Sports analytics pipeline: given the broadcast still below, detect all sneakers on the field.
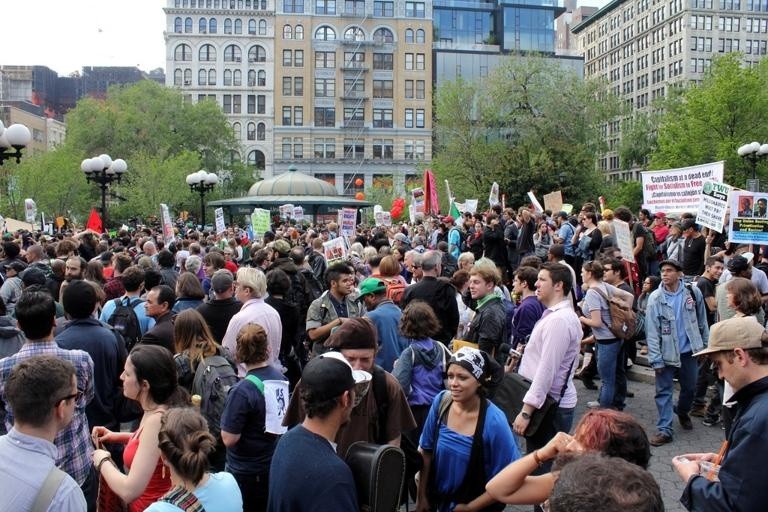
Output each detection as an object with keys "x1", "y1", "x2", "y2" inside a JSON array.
[{"x1": 581, "y1": 378, "x2": 723, "y2": 447}]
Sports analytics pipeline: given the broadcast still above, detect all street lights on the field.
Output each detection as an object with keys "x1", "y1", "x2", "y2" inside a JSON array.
[
  {"x1": 81, "y1": 152, "x2": 130, "y2": 234},
  {"x1": 737, "y1": 140, "x2": 767, "y2": 266},
  {"x1": 186, "y1": 169, "x2": 218, "y2": 228},
  {"x1": 1, "y1": 118, "x2": 30, "y2": 167}
]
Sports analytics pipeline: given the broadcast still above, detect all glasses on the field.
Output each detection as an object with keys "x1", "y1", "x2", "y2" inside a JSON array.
[
  {"x1": 604, "y1": 268, "x2": 614, "y2": 272},
  {"x1": 54, "y1": 390, "x2": 84, "y2": 409}
]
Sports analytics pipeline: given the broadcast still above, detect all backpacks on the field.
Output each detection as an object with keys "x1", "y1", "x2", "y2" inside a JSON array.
[
  {"x1": 592, "y1": 286, "x2": 637, "y2": 340},
  {"x1": 107, "y1": 299, "x2": 143, "y2": 349},
  {"x1": 192, "y1": 353, "x2": 240, "y2": 434}
]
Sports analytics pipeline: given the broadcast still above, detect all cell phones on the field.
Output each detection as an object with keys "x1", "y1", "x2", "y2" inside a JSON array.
[{"x1": 678, "y1": 456, "x2": 690, "y2": 464}]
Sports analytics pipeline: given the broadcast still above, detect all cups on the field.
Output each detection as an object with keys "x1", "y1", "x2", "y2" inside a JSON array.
[{"x1": 699, "y1": 459, "x2": 723, "y2": 482}]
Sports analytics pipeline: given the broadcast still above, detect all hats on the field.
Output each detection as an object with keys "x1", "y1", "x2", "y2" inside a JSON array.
[
  {"x1": 268, "y1": 239, "x2": 291, "y2": 254},
  {"x1": 658, "y1": 259, "x2": 683, "y2": 271},
  {"x1": 5, "y1": 262, "x2": 46, "y2": 288},
  {"x1": 355, "y1": 278, "x2": 385, "y2": 301},
  {"x1": 691, "y1": 318, "x2": 764, "y2": 356},
  {"x1": 302, "y1": 350, "x2": 373, "y2": 393},
  {"x1": 726, "y1": 252, "x2": 755, "y2": 273}
]
